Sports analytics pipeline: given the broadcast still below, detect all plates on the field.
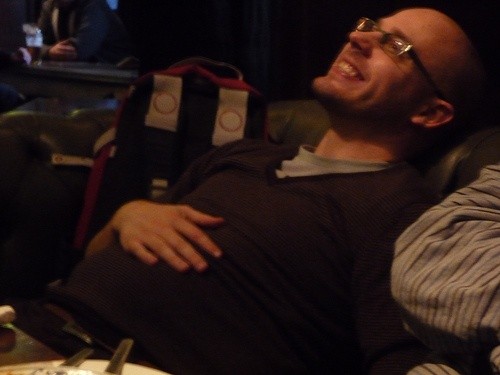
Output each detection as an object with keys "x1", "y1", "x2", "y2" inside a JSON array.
[{"x1": 2, "y1": 359, "x2": 169, "y2": 375}]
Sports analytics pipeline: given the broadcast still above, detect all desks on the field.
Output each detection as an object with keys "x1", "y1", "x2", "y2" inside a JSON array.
[
  {"x1": 0, "y1": 322, "x2": 66, "y2": 374},
  {"x1": 22, "y1": 60, "x2": 138, "y2": 104}
]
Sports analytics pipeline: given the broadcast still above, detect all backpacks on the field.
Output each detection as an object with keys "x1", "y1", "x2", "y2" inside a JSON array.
[{"x1": 76, "y1": 55, "x2": 272, "y2": 253}]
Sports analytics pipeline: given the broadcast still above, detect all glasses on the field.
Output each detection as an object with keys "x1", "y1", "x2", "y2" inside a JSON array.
[{"x1": 355, "y1": 16, "x2": 448, "y2": 103}]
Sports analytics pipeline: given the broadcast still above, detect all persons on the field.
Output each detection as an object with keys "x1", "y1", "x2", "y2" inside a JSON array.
[
  {"x1": 19, "y1": 1, "x2": 486, "y2": 375},
  {"x1": 25, "y1": 0, "x2": 121, "y2": 131}
]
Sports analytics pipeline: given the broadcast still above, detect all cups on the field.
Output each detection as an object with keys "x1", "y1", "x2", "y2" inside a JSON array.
[{"x1": 26, "y1": 36, "x2": 43, "y2": 66}]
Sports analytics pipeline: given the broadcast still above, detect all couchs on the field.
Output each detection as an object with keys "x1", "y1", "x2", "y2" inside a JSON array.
[{"x1": 1, "y1": 103, "x2": 500, "y2": 311}]
